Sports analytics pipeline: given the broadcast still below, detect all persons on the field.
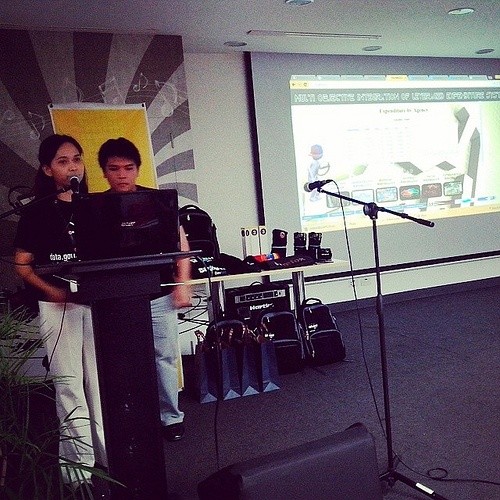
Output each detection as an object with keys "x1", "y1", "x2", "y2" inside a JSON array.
[
  {"x1": 96, "y1": 137, "x2": 193, "y2": 441},
  {"x1": 14, "y1": 133, "x2": 112, "y2": 500}
]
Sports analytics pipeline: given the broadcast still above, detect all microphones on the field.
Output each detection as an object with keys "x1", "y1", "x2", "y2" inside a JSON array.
[
  {"x1": 70, "y1": 175, "x2": 80, "y2": 195},
  {"x1": 304, "y1": 180, "x2": 333, "y2": 192}
]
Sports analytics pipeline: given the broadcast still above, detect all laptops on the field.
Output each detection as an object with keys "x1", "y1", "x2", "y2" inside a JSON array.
[{"x1": 72, "y1": 189, "x2": 182, "y2": 262}]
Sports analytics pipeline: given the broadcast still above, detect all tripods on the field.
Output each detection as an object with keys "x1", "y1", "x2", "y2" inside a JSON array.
[{"x1": 318, "y1": 189, "x2": 449, "y2": 500}]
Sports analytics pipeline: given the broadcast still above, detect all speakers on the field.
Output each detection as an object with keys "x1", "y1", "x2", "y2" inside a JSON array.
[
  {"x1": 198, "y1": 422, "x2": 384, "y2": 500},
  {"x1": 225, "y1": 283, "x2": 289, "y2": 324}
]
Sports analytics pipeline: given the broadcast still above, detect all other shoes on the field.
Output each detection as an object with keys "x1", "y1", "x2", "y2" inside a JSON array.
[
  {"x1": 75, "y1": 482, "x2": 97, "y2": 500},
  {"x1": 162, "y1": 422, "x2": 185, "y2": 441}
]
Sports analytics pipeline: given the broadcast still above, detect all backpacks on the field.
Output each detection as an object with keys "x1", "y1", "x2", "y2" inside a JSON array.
[
  {"x1": 300, "y1": 298, "x2": 346, "y2": 366},
  {"x1": 255, "y1": 302, "x2": 304, "y2": 375},
  {"x1": 178, "y1": 205, "x2": 220, "y2": 279},
  {"x1": 204, "y1": 310, "x2": 248, "y2": 348}
]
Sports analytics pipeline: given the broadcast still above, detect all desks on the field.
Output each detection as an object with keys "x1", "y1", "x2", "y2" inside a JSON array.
[{"x1": 190, "y1": 259, "x2": 349, "y2": 360}]
